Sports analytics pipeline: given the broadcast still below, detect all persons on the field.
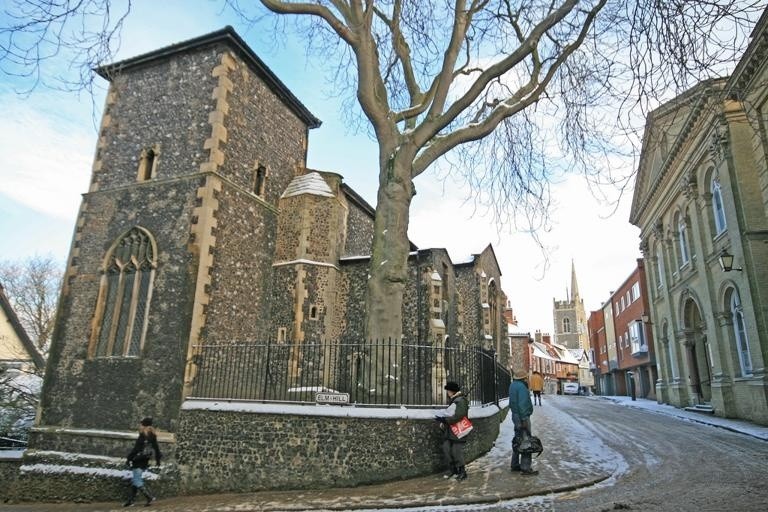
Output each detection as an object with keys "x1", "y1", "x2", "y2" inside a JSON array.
[
  {"x1": 530, "y1": 369, "x2": 545, "y2": 405},
  {"x1": 122, "y1": 416, "x2": 163, "y2": 506},
  {"x1": 434, "y1": 381, "x2": 470, "y2": 482},
  {"x1": 509, "y1": 368, "x2": 540, "y2": 475}
]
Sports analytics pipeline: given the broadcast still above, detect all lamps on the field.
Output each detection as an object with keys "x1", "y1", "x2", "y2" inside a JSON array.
[{"x1": 718, "y1": 247, "x2": 743, "y2": 272}]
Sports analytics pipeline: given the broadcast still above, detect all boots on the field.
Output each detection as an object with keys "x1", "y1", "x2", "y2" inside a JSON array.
[
  {"x1": 124, "y1": 486, "x2": 136, "y2": 507},
  {"x1": 138, "y1": 484, "x2": 155, "y2": 507},
  {"x1": 456, "y1": 466, "x2": 466, "y2": 481},
  {"x1": 449, "y1": 463, "x2": 457, "y2": 479}
]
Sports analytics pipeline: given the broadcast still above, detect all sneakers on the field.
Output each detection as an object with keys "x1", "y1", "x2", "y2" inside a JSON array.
[
  {"x1": 510, "y1": 467, "x2": 520, "y2": 471},
  {"x1": 521, "y1": 469, "x2": 538, "y2": 476}
]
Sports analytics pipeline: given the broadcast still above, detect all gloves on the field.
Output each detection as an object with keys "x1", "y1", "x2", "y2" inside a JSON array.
[{"x1": 434, "y1": 416, "x2": 446, "y2": 423}]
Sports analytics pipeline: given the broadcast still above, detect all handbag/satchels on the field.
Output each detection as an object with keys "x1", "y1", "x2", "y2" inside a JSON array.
[
  {"x1": 132, "y1": 455, "x2": 148, "y2": 469},
  {"x1": 433, "y1": 421, "x2": 449, "y2": 442},
  {"x1": 512, "y1": 436, "x2": 543, "y2": 454}
]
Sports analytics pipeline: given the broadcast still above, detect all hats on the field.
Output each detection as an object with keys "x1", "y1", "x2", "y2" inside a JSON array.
[
  {"x1": 513, "y1": 369, "x2": 528, "y2": 379},
  {"x1": 445, "y1": 382, "x2": 459, "y2": 392},
  {"x1": 141, "y1": 418, "x2": 153, "y2": 426}
]
